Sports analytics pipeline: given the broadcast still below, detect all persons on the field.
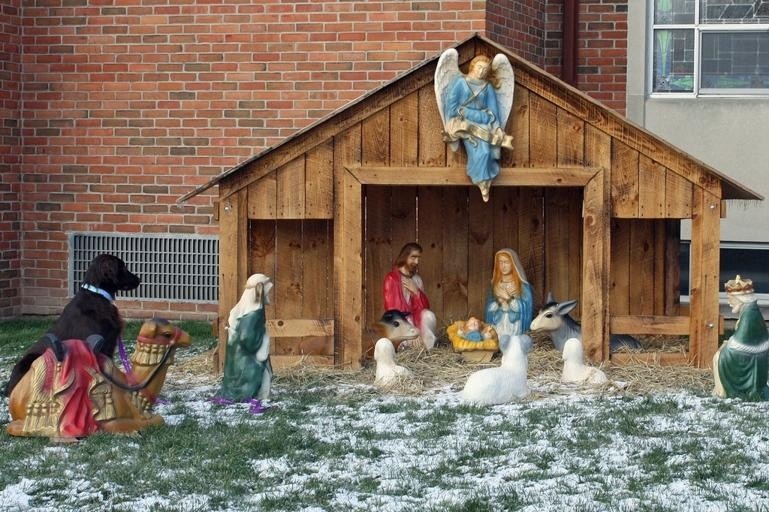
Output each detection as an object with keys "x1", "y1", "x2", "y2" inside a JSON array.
[
  {"x1": 382, "y1": 240, "x2": 439, "y2": 353},
  {"x1": 440, "y1": 54, "x2": 505, "y2": 204},
  {"x1": 459, "y1": 316, "x2": 492, "y2": 342},
  {"x1": 486, "y1": 248, "x2": 535, "y2": 355},
  {"x1": 710, "y1": 298, "x2": 769, "y2": 405},
  {"x1": 210, "y1": 272, "x2": 278, "y2": 407}
]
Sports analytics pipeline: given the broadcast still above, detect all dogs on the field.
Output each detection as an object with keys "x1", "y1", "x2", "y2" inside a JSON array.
[{"x1": 3, "y1": 254, "x2": 143, "y2": 398}]
